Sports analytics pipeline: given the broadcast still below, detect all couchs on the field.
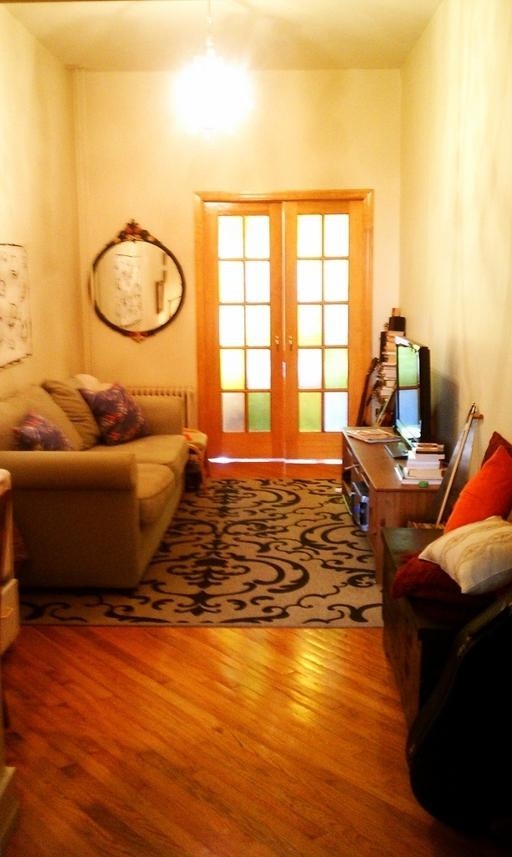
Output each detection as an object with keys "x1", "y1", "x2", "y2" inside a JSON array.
[{"x1": 0, "y1": 384, "x2": 190, "y2": 590}]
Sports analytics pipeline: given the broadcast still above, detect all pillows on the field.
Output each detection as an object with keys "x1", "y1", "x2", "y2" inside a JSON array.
[
  {"x1": 14, "y1": 373, "x2": 141, "y2": 451},
  {"x1": 388, "y1": 443, "x2": 512, "y2": 608}
]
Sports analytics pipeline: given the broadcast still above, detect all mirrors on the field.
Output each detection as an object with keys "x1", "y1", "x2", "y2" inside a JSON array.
[{"x1": 84, "y1": 219, "x2": 185, "y2": 343}]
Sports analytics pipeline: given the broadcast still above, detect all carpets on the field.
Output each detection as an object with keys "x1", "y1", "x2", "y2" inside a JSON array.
[{"x1": 20, "y1": 477, "x2": 385, "y2": 631}]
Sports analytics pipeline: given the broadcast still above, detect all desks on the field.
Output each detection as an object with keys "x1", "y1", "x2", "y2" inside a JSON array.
[{"x1": 339, "y1": 426, "x2": 445, "y2": 553}]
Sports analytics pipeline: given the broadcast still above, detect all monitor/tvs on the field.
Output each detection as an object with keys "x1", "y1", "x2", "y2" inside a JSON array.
[{"x1": 383, "y1": 335, "x2": 431, "y2": 459}]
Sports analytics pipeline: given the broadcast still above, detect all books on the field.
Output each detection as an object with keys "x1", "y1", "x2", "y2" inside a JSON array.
[
  {"x1": 394, "y1": 443, "x2": 445, "y2": 484},
  {"x1": 346, "y1": 429, "x2": 401, "y2": 443},
  {"x1": 381, "y1": 331, "x2": 404, "y2": 395}
]
